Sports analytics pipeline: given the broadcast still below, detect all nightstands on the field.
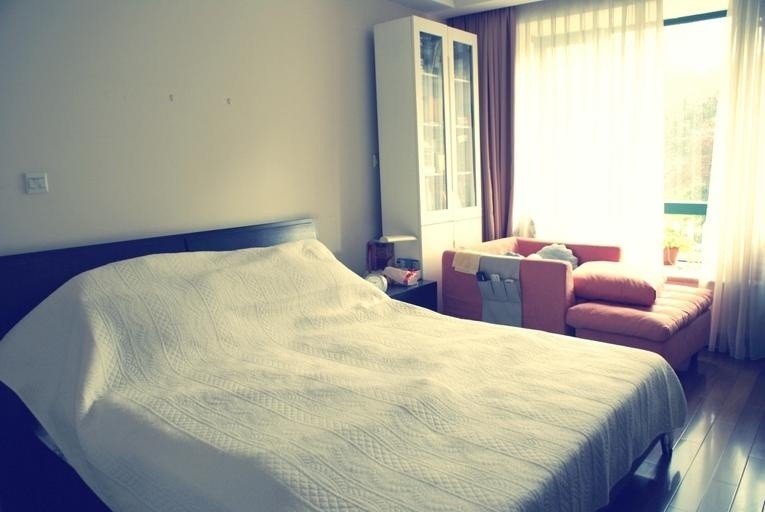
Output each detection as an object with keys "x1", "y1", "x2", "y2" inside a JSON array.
[{"x1": 355, "y1": 270, "x2": 437, "y2": 312}]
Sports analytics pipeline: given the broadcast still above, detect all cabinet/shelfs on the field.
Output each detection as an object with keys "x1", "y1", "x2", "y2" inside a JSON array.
[{"x1": 373, "y1": 14, "x2": 485, "y2": 310}]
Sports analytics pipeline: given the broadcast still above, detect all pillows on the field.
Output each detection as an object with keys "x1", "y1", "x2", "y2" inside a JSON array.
[
  {"x1": 573, "y1": 260, "x2": 666, "y2": 305},
  {"x1": 536, "y1": 243, "x2": 579, "y2": 270}
]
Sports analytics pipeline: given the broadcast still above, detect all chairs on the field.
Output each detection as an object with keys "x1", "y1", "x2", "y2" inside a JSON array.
[{"x1": 442, "y1": 236, "x2": 620, "y2": 345}]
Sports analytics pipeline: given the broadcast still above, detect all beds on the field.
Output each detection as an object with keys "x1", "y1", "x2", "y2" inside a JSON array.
[{"x1": 0, "y1": 215, "x2": 671, "y2": 512}]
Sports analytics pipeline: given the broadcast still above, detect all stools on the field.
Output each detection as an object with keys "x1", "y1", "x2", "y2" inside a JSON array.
[{"x1": 566, "y1": 282, "x2": 714, "y2": 371}]
